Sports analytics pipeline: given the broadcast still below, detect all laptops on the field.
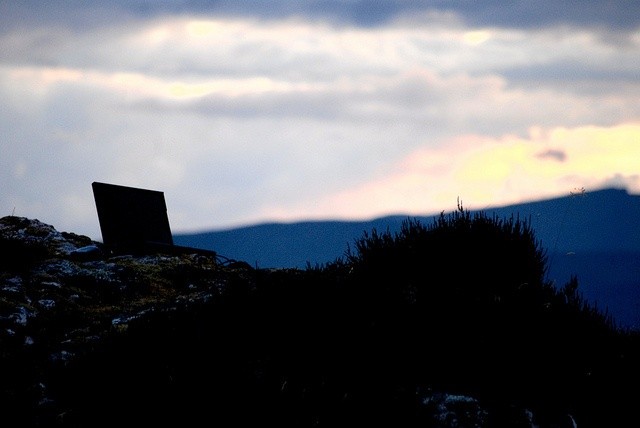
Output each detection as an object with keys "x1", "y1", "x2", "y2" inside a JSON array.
[{"x1": 92, "y1": 182, "x2": 216, "y2": 258}]
